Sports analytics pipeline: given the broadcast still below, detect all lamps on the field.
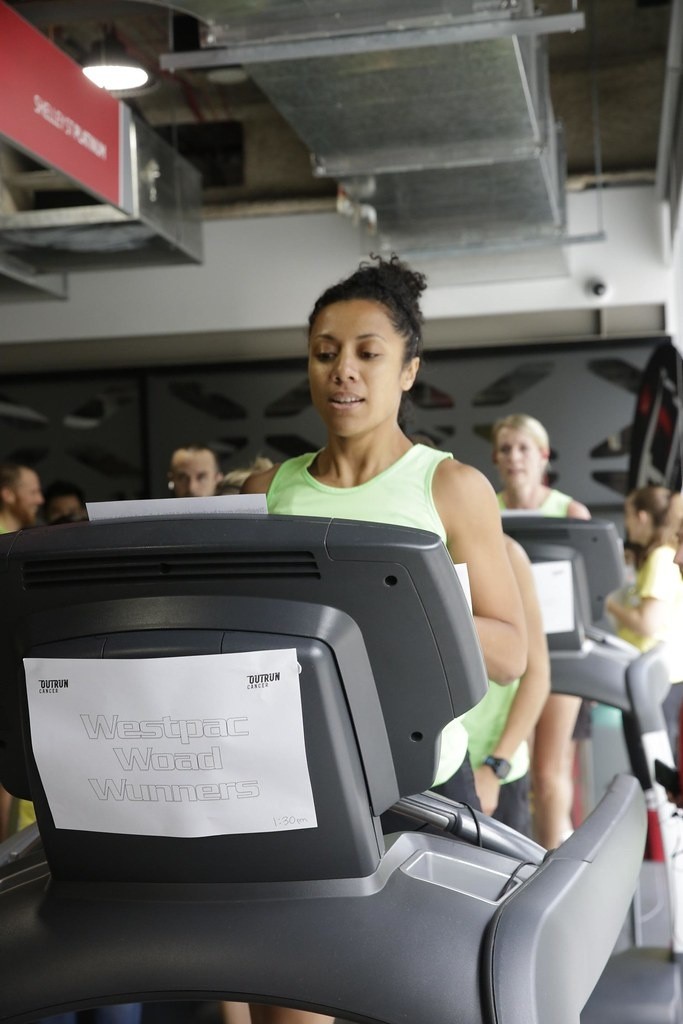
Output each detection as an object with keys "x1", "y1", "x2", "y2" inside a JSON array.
[{"x1": 80, "y1": 20, "x2": 152, "y2": 91}]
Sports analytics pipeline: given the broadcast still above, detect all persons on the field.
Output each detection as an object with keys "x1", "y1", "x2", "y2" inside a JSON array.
[
  {"x1": 221, "y1": 253, "x2": 527, "y2": 1024},
  {"x1": 171, "y1": 447, "x2": 218, "y2": 496},
  {"x1": 411, "y1": 435, "x2": 550, "y2": 839},
  {"x1": 605, "y1": 485, "x2": 683, "y2": 817},
  {"x1": 44, "y1": 480, "x2": 85, "y2": 524},
  {"x1": 0, "y1": 463, "x2": 43, "y2": 841},
  {"x1": 496, "y1": 414, "x2": 597, "y2": 843}
]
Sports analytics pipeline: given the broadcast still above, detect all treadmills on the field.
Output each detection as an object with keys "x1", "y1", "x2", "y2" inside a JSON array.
[{"x1": 0, "y1": 506, "x2": 682, "y2": 1024}]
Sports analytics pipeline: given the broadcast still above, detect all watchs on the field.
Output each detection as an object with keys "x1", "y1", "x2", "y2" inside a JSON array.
[{"x1": 483, "y1": 755, "x2": 512, "y2": 779}]
